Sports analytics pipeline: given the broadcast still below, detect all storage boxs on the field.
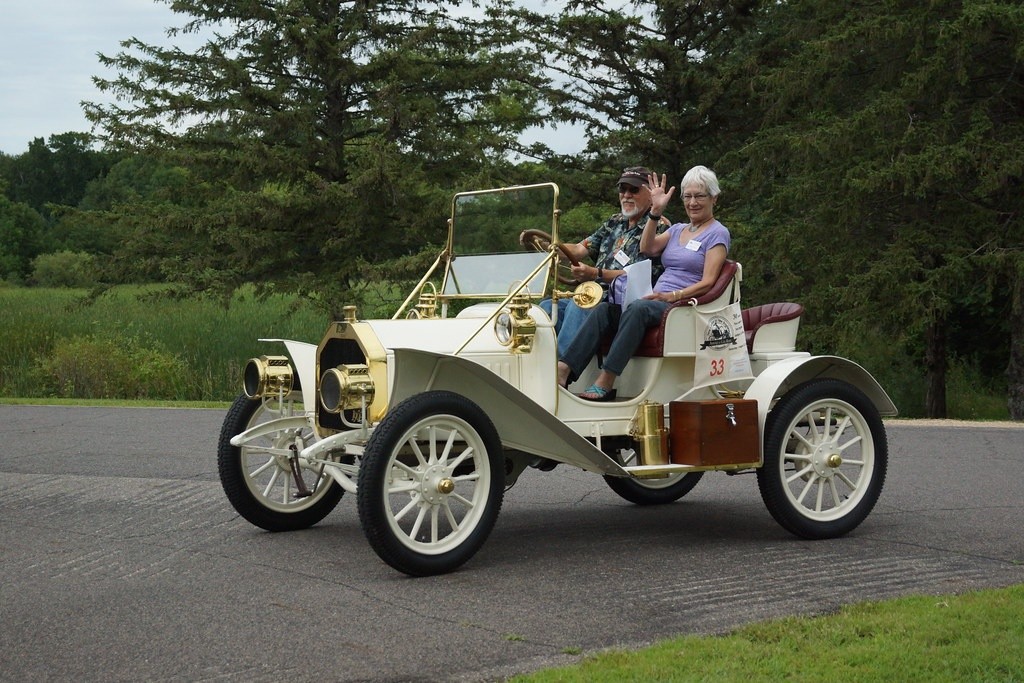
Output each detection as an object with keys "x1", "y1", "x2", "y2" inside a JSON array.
[{"x1": 669, "y1": 397, "x2": 759, "y2": 466}]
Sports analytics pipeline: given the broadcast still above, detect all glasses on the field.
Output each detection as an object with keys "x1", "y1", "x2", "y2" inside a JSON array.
[
  {"x1": 619, "y1": 185, "x2": 640, "y2": 193},
  {"x1": 681, "y1": 193, "x2": 711, "y2": 201}
]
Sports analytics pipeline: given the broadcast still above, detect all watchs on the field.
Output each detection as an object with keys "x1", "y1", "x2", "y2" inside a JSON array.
[{"x1": 595, "y1": 266, "x2": 603, "y2": 283}]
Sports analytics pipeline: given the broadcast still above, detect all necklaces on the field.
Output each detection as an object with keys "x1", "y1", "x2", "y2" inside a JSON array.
[{"x1": 688, "y1": 216, "x2": 715, "y2": 233}]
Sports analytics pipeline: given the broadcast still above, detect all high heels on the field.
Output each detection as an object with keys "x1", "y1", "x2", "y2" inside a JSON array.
[{"x1": 577, "y1": 383, "x2": 617, "y2": 402}]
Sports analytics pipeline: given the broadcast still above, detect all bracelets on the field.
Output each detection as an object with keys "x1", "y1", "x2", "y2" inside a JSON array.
[
  {"x1": 680, "y1": 289, "x2": 684, "y2": 300},
  {"x1": 648, "y1": 212, "x2": 661, "y2": 221},
  {"x1": 672, "y1": 291, "x2": 676, "y2": 301}
]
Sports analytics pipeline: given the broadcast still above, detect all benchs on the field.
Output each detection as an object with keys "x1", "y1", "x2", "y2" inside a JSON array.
[{"x1": 596, "y1": 257, "x2": 742, "y2": 358}]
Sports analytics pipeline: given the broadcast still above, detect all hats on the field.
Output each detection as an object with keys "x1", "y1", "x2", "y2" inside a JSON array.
[{"x1": 615, "y1": 166, "x2": 652, "y2": 188}]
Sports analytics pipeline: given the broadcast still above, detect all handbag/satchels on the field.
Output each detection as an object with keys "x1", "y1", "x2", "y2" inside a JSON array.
[{"x1": 608, "y1": 274, "x2": 627, "y2": 304}]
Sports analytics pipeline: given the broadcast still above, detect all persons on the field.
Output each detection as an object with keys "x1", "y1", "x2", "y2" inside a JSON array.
[{"x1": 519, "y1": 164, "x2": 731, "y2": 401}]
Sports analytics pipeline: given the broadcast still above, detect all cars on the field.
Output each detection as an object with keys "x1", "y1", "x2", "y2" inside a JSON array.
[{"x1": 215, "y1": 182, "x2": 900, "y2": 577}]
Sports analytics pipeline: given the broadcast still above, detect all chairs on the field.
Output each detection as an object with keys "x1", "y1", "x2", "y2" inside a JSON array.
[{"x1": 741, "y1": 303, "x2": 804, "y2": 354}]
